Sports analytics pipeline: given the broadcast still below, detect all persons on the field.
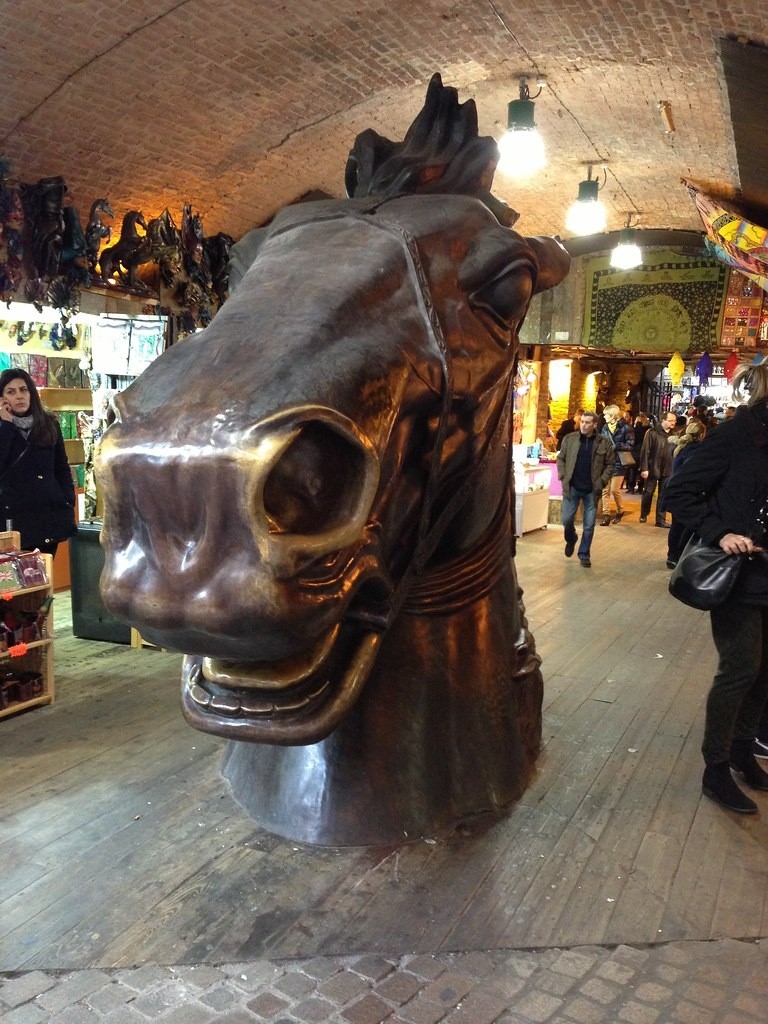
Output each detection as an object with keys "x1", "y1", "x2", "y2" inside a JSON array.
[
  {"x1": 555, "y1": 403, "x2": 737, "y2": 568},
  {"x1": 0, "y1": 368, "x2": 79, "y2": 559},
  {"x1": 156, "y1": 203, "x2": 214, "y2": 339},
  {"x1": 662, "y1": 356, "x2": 768, "y2": 812}
]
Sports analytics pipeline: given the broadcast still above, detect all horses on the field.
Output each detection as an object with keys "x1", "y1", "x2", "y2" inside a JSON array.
[{"x1": 92, "y1": 74, "x2": 571, "y2": 845}]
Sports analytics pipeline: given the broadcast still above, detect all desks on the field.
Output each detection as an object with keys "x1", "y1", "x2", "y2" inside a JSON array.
[
  {"x1": 67, "y1": 522, "x2": 131, "y2": 646},
  {"x1": 0, "y1": 553, "x2": 54, "y2": 718}
]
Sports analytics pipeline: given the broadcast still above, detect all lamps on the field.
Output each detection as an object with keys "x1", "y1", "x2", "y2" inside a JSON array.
[
  {"x1": 566, "y1": 166, "x2": 607, "y2": 235},
  {"x1": 609, "y1": 212, "x2": 643, "y2": 269},
  {"x1": 498, "y1": 84, "x2": 547, "y2": 177}
]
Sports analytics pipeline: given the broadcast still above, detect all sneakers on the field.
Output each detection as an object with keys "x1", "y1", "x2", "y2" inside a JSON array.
[{"x1": 752, "y1": 737, "x2": 768, "y2": 760}]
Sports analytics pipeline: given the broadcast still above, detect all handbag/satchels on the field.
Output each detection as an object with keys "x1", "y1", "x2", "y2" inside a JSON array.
[
  {"x1": 617, "y1": 451, "x2": 636, "y2": 465},
  {"x1": 667, "y1": 532, "x2": 747, "y2": 612}
]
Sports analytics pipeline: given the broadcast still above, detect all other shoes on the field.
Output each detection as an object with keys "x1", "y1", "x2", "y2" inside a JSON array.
[
  {"x1": 625, "y1": 489, "x2": 634, "y2": 494},
  {"x1": 665, "y1": 560, "x2": 677, "y2": 569},
  {"x1": 702, "y1": 761, "x2": 758, "y2": 813},
  {"x1": 634, "y1": 488, "x2": 643, "y2": 495},
  {"x1": 612, "y1": 512, "x2": 624, "y2": 524},
  {"x1": 728, "y1": 740, "x2": 768, "y2": 790},
  {"x1": 600, "y1": 515, "x2": 610, "y2": 526}
]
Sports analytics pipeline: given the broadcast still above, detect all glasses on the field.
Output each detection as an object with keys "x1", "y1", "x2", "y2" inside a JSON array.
[{"x1": 666, "y1": 419, "x2": 677, "y2": 423}]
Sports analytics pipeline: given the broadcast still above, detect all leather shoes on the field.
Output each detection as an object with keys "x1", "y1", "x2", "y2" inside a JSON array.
[
  {"x1": 655, "y1": 521, "x2": 672, "y2": 528},
  {"x1": 565, "y1": 536, "x2": 578, "y2": 557},
  {"x1": 580, "y1": 559, "x2": 591, "y2": 568},
  {"x1": 639, "y1": 513, "x2": 647, "y2": 522}
]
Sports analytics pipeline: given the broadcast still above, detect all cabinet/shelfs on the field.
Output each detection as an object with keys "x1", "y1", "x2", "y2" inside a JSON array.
[
  {"x1": 515, "y1": 465, "x2": 552, "y2": 538},
  {"x1": 33, "y1": 385, "x2": 94, "y2": 594}
]
